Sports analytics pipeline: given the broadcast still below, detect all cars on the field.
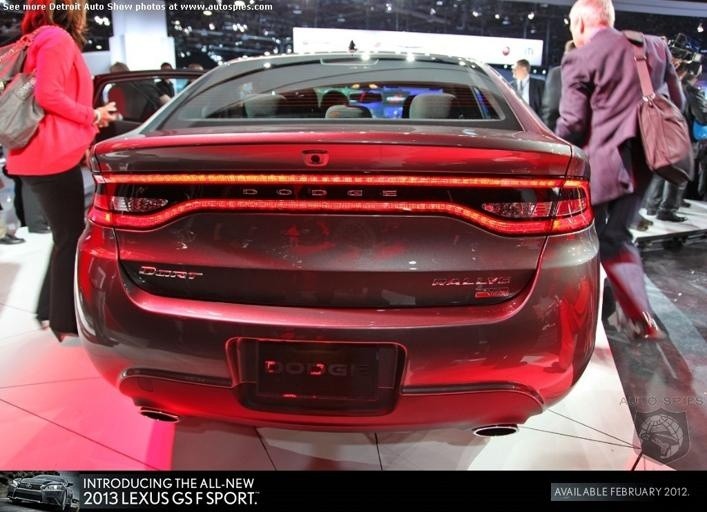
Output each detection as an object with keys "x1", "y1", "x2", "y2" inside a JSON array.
[
  {"x1": 0, "y1": 145, "x2": 98, "y2": 243},
  {"x1": 64, "y1": 42, "x2": 606, "y2": 442}
]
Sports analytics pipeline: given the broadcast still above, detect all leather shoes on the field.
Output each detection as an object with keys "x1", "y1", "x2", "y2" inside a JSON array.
[
  {"x1": 628, "y1": 313, "x2": 661, "y2": 342},
  {"x1": 630, "y1": 198, "x2": 690, "y2": 232},
  {"x1": 0, "y1": 233, "x2": 24, "y2": 245},
  {"x1": 28, "y1": 222, "x2": 52, "y2": 233}
]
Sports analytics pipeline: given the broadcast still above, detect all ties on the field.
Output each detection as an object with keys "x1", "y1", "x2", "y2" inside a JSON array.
[{"x1": 518, "y1": 82, "x2": 523, "y2": 97}]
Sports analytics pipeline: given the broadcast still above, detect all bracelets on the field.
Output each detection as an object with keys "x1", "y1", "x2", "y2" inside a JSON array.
[{"x1": 92, "y1": 110, "x2": 102, "y2": 126}]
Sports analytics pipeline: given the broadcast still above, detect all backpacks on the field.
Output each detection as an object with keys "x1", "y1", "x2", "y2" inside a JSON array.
[{"x1": 636, "y1": 95, "x2": 694, "y2": 183}]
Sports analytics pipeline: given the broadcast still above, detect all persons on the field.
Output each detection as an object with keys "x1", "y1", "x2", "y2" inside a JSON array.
[
  {"x1": 0, "y1": 145, "x2": 25, "y2": 246},
  {"x1": 553, "y1": 0, "x2": 688, "y2": 339},
  {"x1": 107, "y1": 63, "x2": 151, "y2": 124},
  {"x1": 16, "y1": 175, "x2": 52, "y2": 234},
  {"x1": 507, "y1": 59, "x2": 547, "y2": 123},
  {"x1": 0, "y1": 1, "x2": 121, "y2": 345},
  {"x1": 628, "y1": 33, "x2": 706, "y2": 231},
  {"x1": 154, "y1": 63, "x2": 176, "y2": 108},
  {"x1": 183, "y1": 63, "x2": 205, "y2": 90}
]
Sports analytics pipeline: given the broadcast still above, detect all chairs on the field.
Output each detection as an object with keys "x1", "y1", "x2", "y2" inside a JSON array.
[{"x1": 200, "y1": 93, "x2": 461, "y2": 119}]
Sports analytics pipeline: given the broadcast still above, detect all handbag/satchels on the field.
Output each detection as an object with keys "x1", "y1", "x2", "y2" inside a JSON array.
[{"x1": 0, "y1": 73, "x2": 45, "y2": 151}]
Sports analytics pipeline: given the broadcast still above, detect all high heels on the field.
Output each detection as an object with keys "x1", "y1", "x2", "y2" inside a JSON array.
[
  {"x1": 49, "y1": 320, "x2": 82, "y2": 341},
  {"x1": 36, "y1": 314, "x2": 60, "y2": 330}
]
